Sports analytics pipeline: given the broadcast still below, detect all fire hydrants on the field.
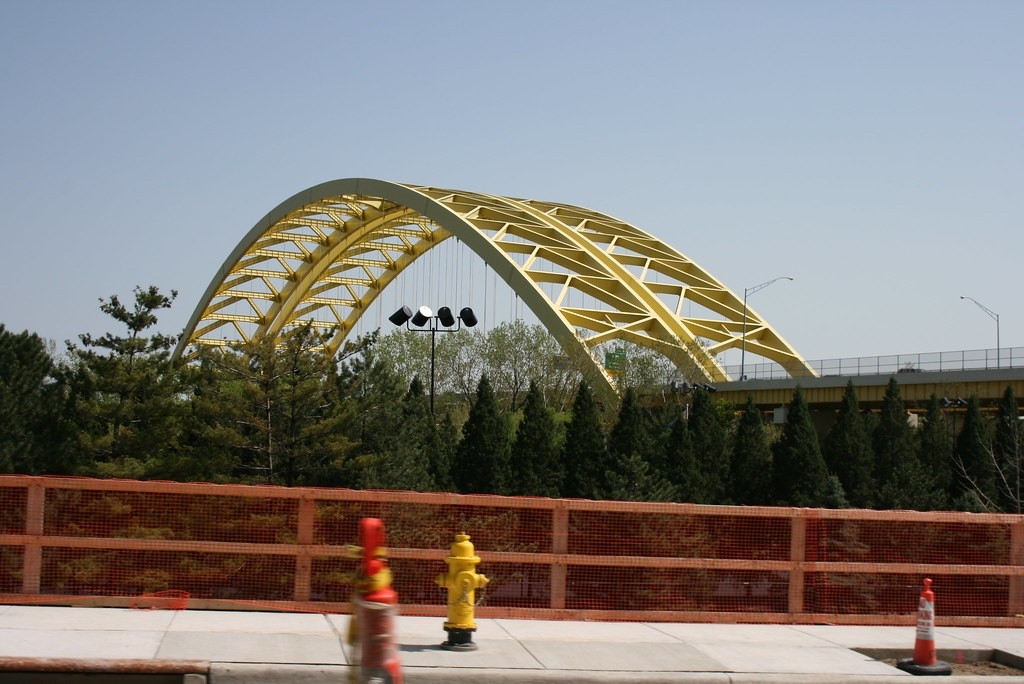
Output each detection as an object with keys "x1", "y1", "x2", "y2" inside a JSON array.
[{"x1": 431, "y1": 529, "x2": 492, "y2": 653}]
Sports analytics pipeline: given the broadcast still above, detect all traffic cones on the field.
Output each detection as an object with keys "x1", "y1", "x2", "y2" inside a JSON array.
[{"x1": 895, "y1": 577, "x2": 953, "y2": 676}]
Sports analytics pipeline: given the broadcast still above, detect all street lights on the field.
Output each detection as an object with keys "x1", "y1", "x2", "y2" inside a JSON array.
[
  {"x1": 388, "y1": 305, "x2": 478, "y2": 412},
  {"x1": 739, "y1": 275, "x2": 795, "y2": 382},
  {"x1": 960, "y1": 295, "x2": 1000, "y2": 368}
]
